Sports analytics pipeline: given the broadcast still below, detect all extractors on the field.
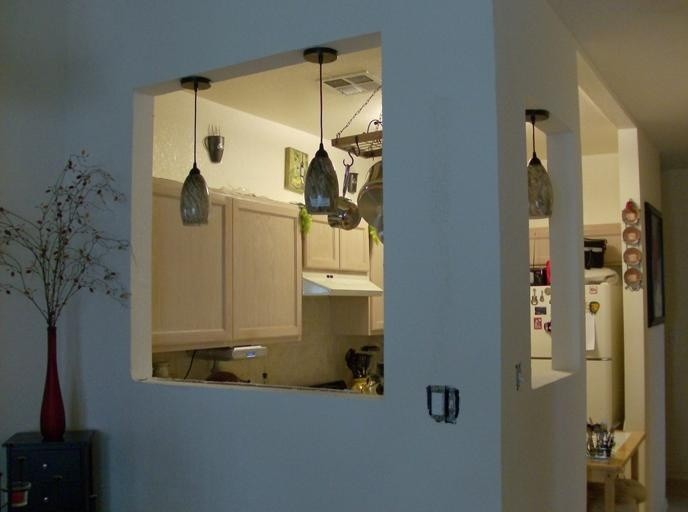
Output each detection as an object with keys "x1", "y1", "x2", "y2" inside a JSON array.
[{"x1": 304, "y1": 274, "x2": 383, "y2": 298}]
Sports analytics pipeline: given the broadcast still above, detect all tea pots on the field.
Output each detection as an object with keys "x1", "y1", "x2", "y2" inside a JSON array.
[{"x1": 348, "y1": 375, "x2": 371, "y2": 392}]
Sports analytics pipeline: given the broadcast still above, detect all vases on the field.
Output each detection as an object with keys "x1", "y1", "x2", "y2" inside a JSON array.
[{"x1": 39, "y1": 328, "x2": 66, "y2": 442}]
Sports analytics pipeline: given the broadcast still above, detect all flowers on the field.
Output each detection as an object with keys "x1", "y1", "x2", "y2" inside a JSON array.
[{"x1": 0, "y1": 148, "x2": 135, "y2": 328}]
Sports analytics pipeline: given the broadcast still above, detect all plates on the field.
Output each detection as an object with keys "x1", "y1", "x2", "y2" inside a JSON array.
[
  {"x1": 622, "y1": 209, "x2": 641, "y2": 224},
  {"x1": 623, "y1": 248, "x2": 642, "y2": 265},
  {"x1": 624, "y1": 269, "x2": 641, "y2": 286},
  {"x1": 623, "y1": 226, "x2": 640, "y2": 244}
]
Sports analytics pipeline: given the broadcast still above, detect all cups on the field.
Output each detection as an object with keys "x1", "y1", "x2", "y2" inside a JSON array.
[{"x1": 349, "y1": 173, "x2": 358, "y2": 193}]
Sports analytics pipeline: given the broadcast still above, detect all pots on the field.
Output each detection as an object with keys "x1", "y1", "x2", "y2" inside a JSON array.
[
  {"x1": 357, "y1": 161, "x2": 383, "y2": 229},
  {"x1": 328, "y1": 165, "x2": 363, "y2": 230}
]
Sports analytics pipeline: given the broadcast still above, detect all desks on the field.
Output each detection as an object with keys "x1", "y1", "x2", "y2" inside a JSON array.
[{"x1": 586, "y1": 430, "x2": 647, "y2": 512}]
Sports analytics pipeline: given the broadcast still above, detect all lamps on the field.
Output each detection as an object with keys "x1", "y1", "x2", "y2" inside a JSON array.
[
  {"x1": 179, "y1": 77, "x2": 211, "y2": 228},
  {"x1": 524, "y1": 107, "x2": 554, "y2": 221},
  {"x1": 301, "y1": 47, "x2": 340, "y2": 214}
]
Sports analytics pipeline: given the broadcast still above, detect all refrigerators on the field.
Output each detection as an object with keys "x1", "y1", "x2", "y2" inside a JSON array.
[{"x1": 530, "y1": 284, "x2": 622, "y2": 431}]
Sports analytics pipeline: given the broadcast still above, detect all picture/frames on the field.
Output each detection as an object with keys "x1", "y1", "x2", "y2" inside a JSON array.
[{"x1": 644, "y1": 199, "x2": 667, "y2": 328}]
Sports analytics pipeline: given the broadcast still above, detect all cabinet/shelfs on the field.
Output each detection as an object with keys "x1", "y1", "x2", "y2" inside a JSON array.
[
  {"x1": 149, "y1": 175, "x2": 304, "y2": 355},
  {"x1": 3, "y1": 428, "x2": 97, "y2": 512},
  {"x1": 302, "y1": 204, "x2": 371, "y2": 276},
  {"x1": 331, "y1": 226, "x2": 384, "y2": 338}
]
